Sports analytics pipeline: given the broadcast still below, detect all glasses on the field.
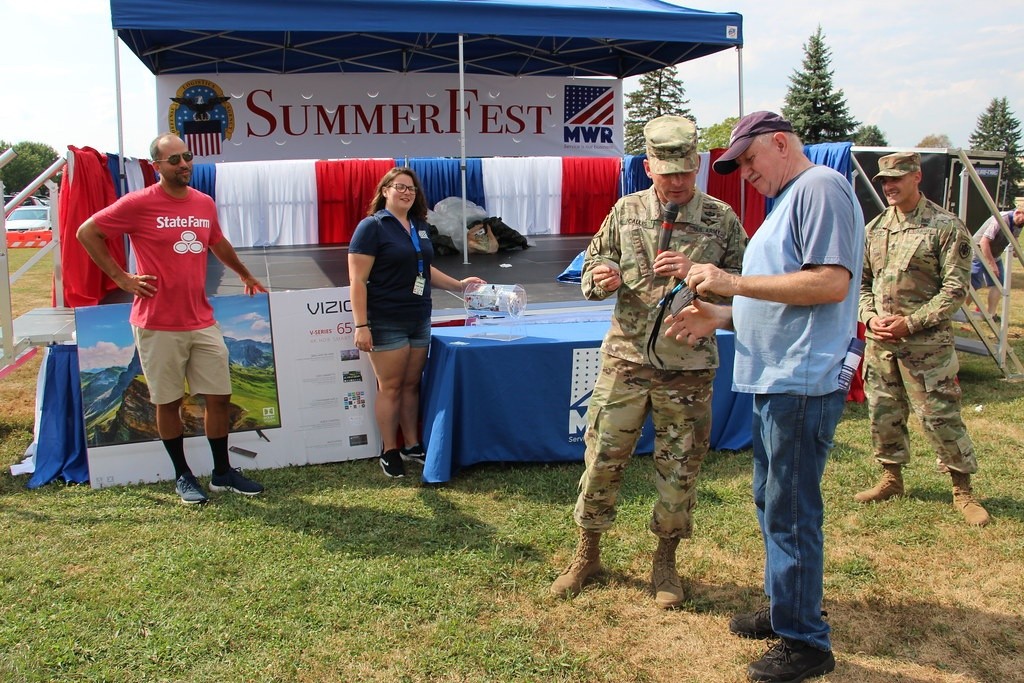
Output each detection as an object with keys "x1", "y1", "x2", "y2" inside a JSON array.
[
  {"x1": 388, "y1": 183, "x2": 418, "y2": 195},
  {"x1": 157, "y1": 151, "x2": 193, "y2": 165}
]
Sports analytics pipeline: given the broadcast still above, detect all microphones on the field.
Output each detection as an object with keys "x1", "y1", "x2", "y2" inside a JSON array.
[{"x1": 657, "y1": 201, "x2": 679, "y2": 257}]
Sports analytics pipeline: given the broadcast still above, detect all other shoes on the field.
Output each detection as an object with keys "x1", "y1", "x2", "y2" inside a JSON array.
[{"x1": 984, "y1": 313, "x2": 1001, "y2": 323}]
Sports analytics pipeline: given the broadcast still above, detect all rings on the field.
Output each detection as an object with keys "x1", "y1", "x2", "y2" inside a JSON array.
[{"x1": 671, "y1": 264, "x2": 675, "y2": 271}]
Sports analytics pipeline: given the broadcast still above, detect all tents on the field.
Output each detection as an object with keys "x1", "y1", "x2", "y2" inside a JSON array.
[
  {"x1": 109, "y1": 0, "x2": 744, "y2": 273},
  {"x1": 348, "y1": 166, "x2": 487, "y2": 478}
]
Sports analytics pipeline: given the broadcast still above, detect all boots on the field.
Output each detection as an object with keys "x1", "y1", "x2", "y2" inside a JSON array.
[
  {"x1": 855, "y1": 464, "x2": 904, "y2": 503},
  {"x1": 551, "y1": 526, "x2": 602, "y2": 598},
  {"x1": 648, "y1": 537, "x2": 685, "y2": 609},
  {"x1": 951, "y1": 470, "x2": 990, "y2": 527}
]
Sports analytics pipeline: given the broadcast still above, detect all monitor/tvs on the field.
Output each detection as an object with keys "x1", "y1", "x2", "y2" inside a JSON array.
[{"x1": 75, "y1": 293, "x2": 282, "y2": 442}]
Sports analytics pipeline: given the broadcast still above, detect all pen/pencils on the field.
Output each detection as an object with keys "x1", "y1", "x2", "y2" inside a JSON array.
[
  {"x1": 656, "y1": 278, "x2": 687, "y2": 309},
  {"x1": 478, "y1": 315, "x2": 506, "y2": 319}
]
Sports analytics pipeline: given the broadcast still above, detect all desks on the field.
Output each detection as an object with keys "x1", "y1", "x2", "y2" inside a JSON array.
[{"x1": 420, "y1": 297, "x2": 753, "y2": 482}]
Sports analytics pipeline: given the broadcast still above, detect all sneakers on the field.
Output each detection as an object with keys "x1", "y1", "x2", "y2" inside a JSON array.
[
  {"x1": 400, "y1": 444, "x2": 426, "y2": 465},
  {"x1": 748, "y1": 636, "x2": 836, "y2": 683},
  {"x1": 208, "y1": 467, "x2": 265, "y2": 495},
  {"x1": 379, "y1": 448, "x2": 405, "y2": 479},
  {"x1": 729, "y1": 606, "x2": 828, "y2": 639},
  {"x1": 175, "y1": 472, "x2": 206, "y2": 504}
]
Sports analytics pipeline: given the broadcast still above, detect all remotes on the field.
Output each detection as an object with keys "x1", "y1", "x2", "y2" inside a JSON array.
[{"x1": 229, "y1": 446, "x2": 257, "y2": 458}]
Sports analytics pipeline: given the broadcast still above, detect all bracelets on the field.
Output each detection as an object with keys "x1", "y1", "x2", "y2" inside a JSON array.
[
  {"x1": 461, "y1": 280, "x2": 464, "y2": 293},
  {"x1": 356, "y1": 323, "x2": 368, "y2": 328}
]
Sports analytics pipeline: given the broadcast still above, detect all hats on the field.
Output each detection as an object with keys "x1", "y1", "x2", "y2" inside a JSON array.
[
  {"x1": 712, "y1": 111, "x2": 794, "y2": 175},
  {"x1": 643, "y1": 114, "x2": 699, "y2": 175},
  {"x1": 872, "y1": 152, "x2": 922, "y2": 181}
]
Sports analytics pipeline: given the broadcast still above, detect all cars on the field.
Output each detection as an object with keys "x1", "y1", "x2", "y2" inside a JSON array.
[
  {"x1": 4, "y1": 193, "x2": 49, "y2": 206},
  {"x1": 5, "y1": 206, "x2": 52, "y2": 232}
]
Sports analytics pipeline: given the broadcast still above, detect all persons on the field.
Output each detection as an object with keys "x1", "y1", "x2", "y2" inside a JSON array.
[
  {"x1": 966, "y1": 197, "x2": 1024, "y2": 323},
  {"x1": 687, "y1": 111, "x2": 866, "y2": 683},
  {"x1": 551, "y1": 116, "x2": 750, "y2": 609},
  {"x1": 76, "y1": 132, "x2": 268, "y2": 504},
  {"x1": 858, "y1": 152, "x2": 990, "y2": 526}
]
